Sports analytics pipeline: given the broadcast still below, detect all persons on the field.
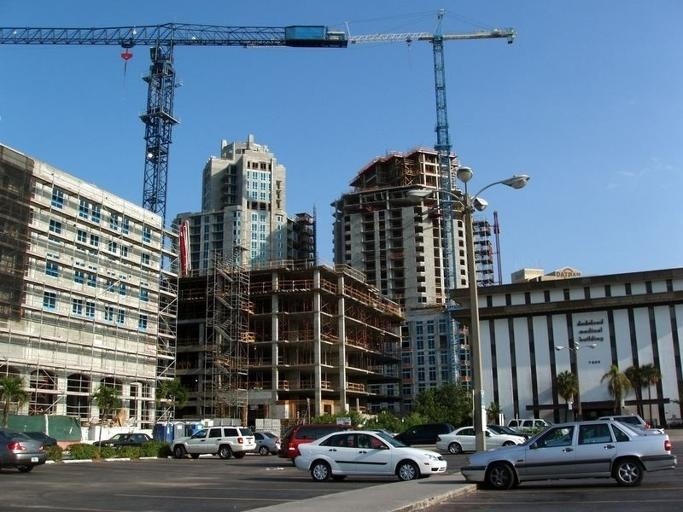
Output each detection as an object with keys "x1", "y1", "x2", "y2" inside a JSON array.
[{"x1": 360, "y1": 436, "x2": 374, "y2": 448}]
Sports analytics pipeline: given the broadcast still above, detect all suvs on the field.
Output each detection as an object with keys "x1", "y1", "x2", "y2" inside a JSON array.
[
  {"x1": 279, "y1": 425, "x2": 387, "y2": 466},
  {"x1": 393, "y1": 423, "x2": 455, "y2": 447},
  {"x1": 506, "y1": 419, "x2": 549, "y2": 433},
  {"x1": 170, "y1": 426, "x2": 257, "y2": 460}
]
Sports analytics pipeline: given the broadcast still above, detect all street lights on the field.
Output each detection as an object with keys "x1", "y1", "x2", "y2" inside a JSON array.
[
  {"x1": 406, "y1": 165, "x2": 530, "y2": 452},
  {"x1": 555, "y1": 342, "x2": 597, "y2": 422}
]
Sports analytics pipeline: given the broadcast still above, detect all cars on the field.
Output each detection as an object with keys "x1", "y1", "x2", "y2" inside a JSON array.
[
  {"x1": 460, "y1": 420, "x2": 678, "y2": 490},
  {"x1": 246, "y1": 431, "x2": 282, "y2": 457},
  {"x1": 547, "y1": 415, "x2": 666, "y2": 447},
  {"x1": 0, "y1": 428, "x2": 57, "y2": 473},
  {"x1": 295, "y1": 430, "x2": 447, "y2": 483},
  {"x1": 438, "y1": 425, "x2": 527, "y2": 454},
  {"x1": 93, "y1": 433, "x2": 153, "y2": 448},
  {"x1": 487, "y1": 425, "x2": 532, "y2": 440}
]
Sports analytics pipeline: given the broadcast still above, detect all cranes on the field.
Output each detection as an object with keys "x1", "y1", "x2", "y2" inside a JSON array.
[
  {"x1": 0, "y1": 23, "x2": 348, "y2": 285},
  {"x1": 327, "y1": 8, "x2": 517, "y2": 384}
]
